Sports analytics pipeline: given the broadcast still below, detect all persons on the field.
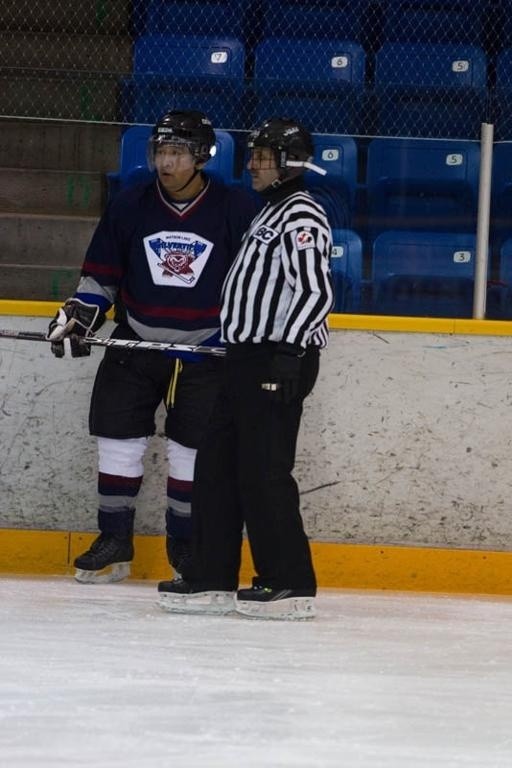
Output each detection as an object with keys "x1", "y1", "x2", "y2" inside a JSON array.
[
  {"x1": 46, "y1": 110, "x2": 262, "y2": 572},
  {"x1": 158, "y1": 114, "x2": 335, "y2": 601}
]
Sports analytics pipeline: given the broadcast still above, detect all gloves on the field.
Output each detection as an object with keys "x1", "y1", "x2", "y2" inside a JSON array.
[
  {"x1": 45, "y1": 297, "x2": 106, "y2": 359},
  {"x1": 260, "y1": 340, "x2": 306, "y2": 406}
]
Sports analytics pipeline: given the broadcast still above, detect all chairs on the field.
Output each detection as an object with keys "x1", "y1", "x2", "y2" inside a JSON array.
[{"x1": 100, "y1": 2, "x2": 510, "y2": 318}]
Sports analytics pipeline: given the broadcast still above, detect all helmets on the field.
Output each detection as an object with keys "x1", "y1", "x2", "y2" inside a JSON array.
[
  {"x1": 242, "y1": 116, "x2": 315, "y2": 179},
  {"x1": 144, "y1": 109, "x2": 216, "y2": 175}
]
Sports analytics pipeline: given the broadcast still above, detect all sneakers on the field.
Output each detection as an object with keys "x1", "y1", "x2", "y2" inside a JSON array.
[
  {"x1": 73, "y1": 531, "x2": 134, "y2": 571},
  {"x1": 165, "y1": 534, "x2": 193, "y2": 575},
  {"x1": 158, "y1": 576, "x2": 239, "y2": 594},
  {"x1": 237, "y1": 584, "x2": 316, "y2": 602}
]
